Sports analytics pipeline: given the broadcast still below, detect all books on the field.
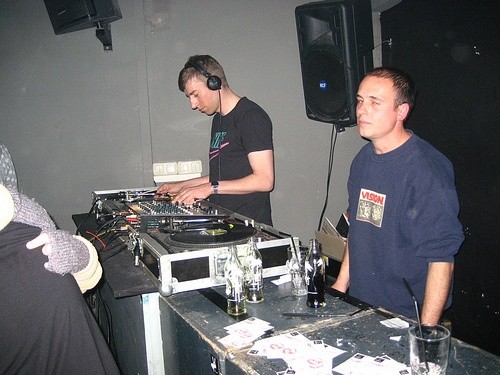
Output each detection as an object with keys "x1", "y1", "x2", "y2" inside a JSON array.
[{"x1": 320, "y1": 212, "x2": 350, "y2": 240}]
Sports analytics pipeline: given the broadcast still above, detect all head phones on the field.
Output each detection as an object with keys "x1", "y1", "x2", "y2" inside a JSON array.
[{"x1": 187, "y1": 60, "x2": 221, "y2": 90}]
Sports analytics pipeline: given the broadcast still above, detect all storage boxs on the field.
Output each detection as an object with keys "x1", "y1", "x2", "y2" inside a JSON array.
[{"x1": 314, "y1": 230, "x2": 348, "y2": 261}]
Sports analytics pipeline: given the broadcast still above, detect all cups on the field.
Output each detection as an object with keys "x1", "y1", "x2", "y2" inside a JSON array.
[
  {"x1": 408, "y1": 323, "x2": 451, "y2": 375},
  {"x1": 287, "y1": 246, "x2": 310, "y2": 296}
]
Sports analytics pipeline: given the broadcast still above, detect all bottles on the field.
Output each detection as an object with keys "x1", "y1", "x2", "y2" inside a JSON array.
[
  {"x1": 304, "y1": 239, "x2": 326, "y2": 308},
  {"x1": 243, "y1": 236, "x2": 265, "y2": 303},
  {"x1": 224, "y1": 245, "x2": 247, "y2": 316}
]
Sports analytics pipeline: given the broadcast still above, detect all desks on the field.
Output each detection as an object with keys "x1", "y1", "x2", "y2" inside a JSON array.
[{"x1": 160, "y1": 277, "x2": 500, "y2": 375}]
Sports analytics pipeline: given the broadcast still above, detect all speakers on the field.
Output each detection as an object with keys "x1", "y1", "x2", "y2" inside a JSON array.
[
  {"x1": 43, "y1": 0, "x2": 121, "y2": 35},
  {"x1": 294, "y1": 0, "x2": 374, "y2": 126}
]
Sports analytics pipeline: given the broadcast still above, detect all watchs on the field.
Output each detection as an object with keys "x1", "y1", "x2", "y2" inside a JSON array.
[{"x1": 211, "y1": 180, "x2": 219, "y2": 194}]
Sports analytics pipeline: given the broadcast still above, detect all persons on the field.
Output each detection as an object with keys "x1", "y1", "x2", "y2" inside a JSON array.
[
  {"x1": 154, "y1": 55, "x2": 274, "y2": 227},
  {"x1": 0, "y1": 142, "x2": 118, "y2": 375},
  {"x1": 329, "y1": 67, "x2": 465, "y2": 327}
]
